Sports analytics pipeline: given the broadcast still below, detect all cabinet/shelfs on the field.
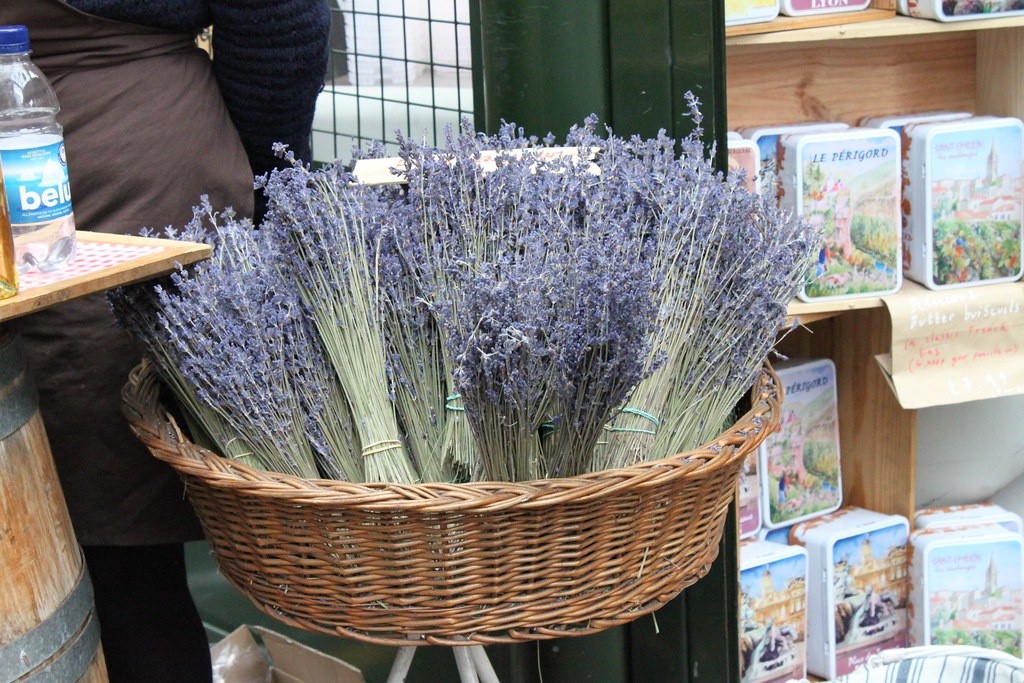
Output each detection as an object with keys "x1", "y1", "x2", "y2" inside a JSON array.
[{"x1": 723, "y1": 0, "x2": 1024, "y2": 683}]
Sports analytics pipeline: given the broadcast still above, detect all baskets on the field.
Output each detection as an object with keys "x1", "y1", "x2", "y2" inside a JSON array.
[{"x1": 119, "y1": 352, "x2": 785, "y2": 646}]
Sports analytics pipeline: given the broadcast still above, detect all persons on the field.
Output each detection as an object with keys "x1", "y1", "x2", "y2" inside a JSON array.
[{"x1": 0, "y1": 0, "x2": 332, "y2": 683}]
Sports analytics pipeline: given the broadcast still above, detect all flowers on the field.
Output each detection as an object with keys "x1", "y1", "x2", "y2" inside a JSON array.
[{"x1": 105, "y1": 90, "x2": 836, "y2": 482}]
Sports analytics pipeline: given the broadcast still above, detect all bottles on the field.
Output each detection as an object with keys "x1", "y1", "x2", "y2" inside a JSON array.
[
  {"x1": 0, "y1": 160, "x2": 20, "y2": 300},
  {"x1": 0, "y1": 24, "x2": 77, "y2": 276}
]
